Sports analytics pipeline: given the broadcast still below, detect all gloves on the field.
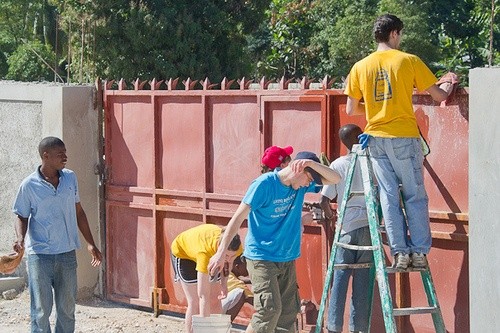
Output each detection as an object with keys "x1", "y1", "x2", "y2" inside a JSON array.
[
  {"x1": 437, "y1": 71, "x2": 458, "y2": 89},
  {"x1": 358, "y1": 134, "x2": 372, "y2": 150}
]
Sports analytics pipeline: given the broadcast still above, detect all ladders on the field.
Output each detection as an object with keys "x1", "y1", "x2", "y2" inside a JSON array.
[{"x1": 314, "y1": 145, "x2": 447, "y2": 333}]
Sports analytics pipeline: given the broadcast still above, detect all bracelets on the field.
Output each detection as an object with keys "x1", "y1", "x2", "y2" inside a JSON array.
[{"x1": 325, "y1": 211, "x2": 336, "y2": 220}]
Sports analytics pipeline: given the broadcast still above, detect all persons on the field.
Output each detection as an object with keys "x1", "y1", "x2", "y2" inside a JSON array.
[
  {"x1": 208, "y1": 151, "x2": 342, "y2": 333},
  {"x1": 217, "y1": 256, "x2": 313, "y2": 326},
  {"x1": 10, "y1": 136, "x2": 103, "y2": 332},
  {"x1": 170, "y1": 224, "x2": 243, "y2": 333},
  {"x1": 259, "y1": 145, "x2": 294, "y2": 177},
  {"x1": 344, "y1": 12, "x2": 460, "y2": 271},
  {"x1": 318, "y1": 124, "x2": 383, "y2": 333}
]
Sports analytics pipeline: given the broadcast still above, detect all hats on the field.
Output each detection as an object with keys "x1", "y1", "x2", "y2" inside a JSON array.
[
  {"x1": 262, "y1": 146, "x2": 293, "y2": 170},
  {"x1": 294, "y1": 151, "x2": 323, "y2": 185}
]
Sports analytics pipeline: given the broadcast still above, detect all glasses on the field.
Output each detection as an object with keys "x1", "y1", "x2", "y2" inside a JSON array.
[
  {"x1": 282, "y1": 156, "x2": 291, "y2": 162},
  {"x1": 305, "y1": 171, "x2": 313, "y2": 183}
]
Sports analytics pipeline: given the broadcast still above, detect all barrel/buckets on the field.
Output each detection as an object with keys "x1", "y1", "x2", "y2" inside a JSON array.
[{"x1": 192, "y1": 314, "x2": 231, "y2": 333}]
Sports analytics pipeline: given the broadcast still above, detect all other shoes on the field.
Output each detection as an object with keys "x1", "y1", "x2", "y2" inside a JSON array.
[
  {"x1": 394, "y1": 252, "x2": 410, "y2": 271},
  {"x1": 412, "y1": 252, "x2": 428, "y2": 271}
]
